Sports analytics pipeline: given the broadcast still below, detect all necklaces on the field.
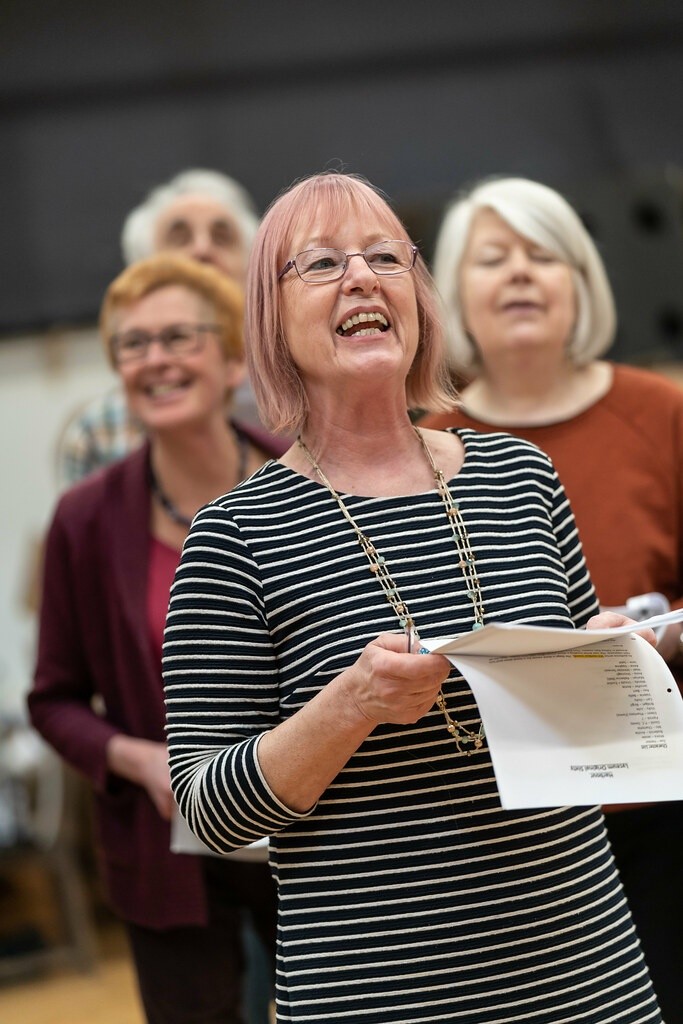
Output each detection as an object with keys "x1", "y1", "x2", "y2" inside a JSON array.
[
  {"x1": 298, "y1": 425, "x2": 487, "y2": 758},
  {"x1": 145, "y1": 426, "x2": 250, "y2": 532}
]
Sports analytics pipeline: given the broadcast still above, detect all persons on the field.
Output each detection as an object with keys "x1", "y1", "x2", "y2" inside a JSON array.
[{"x1": 23, "y1": 167, "x2": 683, "y2": 1024}]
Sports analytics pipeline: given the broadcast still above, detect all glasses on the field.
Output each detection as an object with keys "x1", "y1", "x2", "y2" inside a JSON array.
[
  {"x1": 109, "y1": 322, "x2": 224, "y2": 362},
  {"x1": 278, "y1": 240, "x2": 419, "y2": 283}
]
